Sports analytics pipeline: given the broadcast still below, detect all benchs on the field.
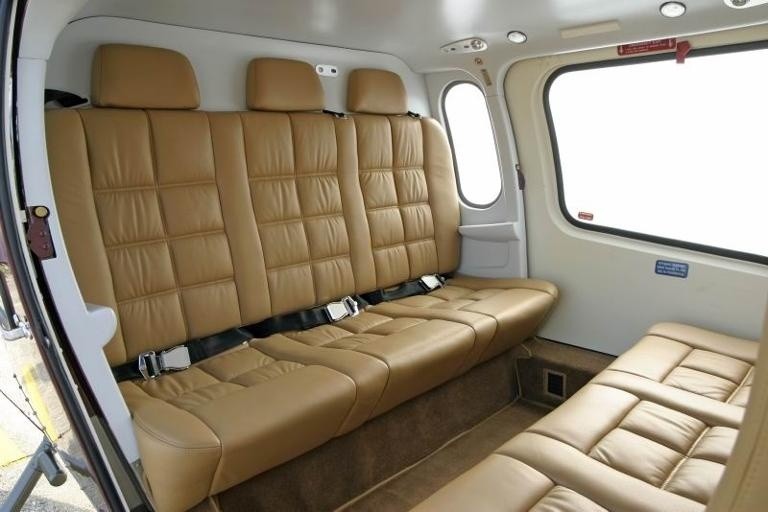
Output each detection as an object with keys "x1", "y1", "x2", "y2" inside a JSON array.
[
  {"x1": 45, "y1": 45, "x2": 558, "y2": 510},
  {"x1": 407, "y1": 299, "x2": 768, "y2": 512}
]
